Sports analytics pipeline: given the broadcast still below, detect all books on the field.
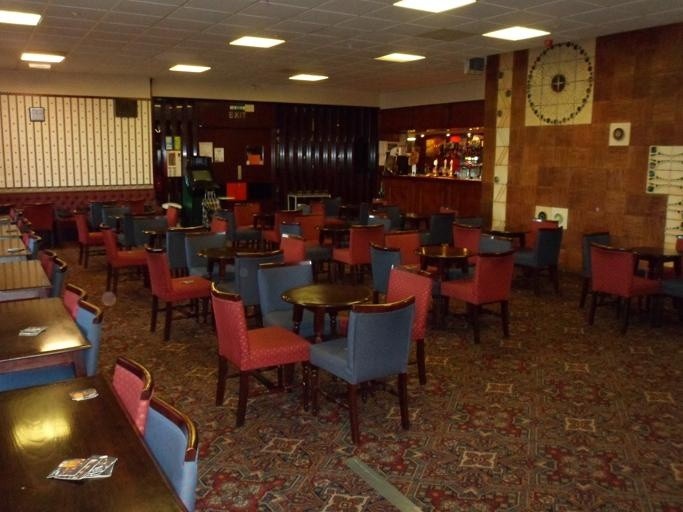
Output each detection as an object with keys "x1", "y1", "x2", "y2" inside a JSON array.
[
  {"x1": 55, "y1": 456, "x2": 90, "y2": 476},
  {"x1": 0, "y1": 217, "x2": 11, "y2": 224},
  {"x1": 19, "y1": 323, "x2": 49, "y2": 338},
  {"x1": 7, "y1": 244, "x2": 27, "y2": 252},
  {"x1": 103, "y1": 464, "x2": 114, "y2": 477},
  {"x1": 66, "y1": 385, "x2": 99, "y2": 402},
  {"x1": 51, "y1": 455, "x2": 119, "y2": 478}
]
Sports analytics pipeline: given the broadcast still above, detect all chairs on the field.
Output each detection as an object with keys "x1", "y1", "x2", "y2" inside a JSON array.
[
  {"x1": 143, "y1": 395, "x2": 198, "y2": 512},
  {"x1": 40, "y1": 249, "x2": 56, "y2": 281},
  {"x1": 63, "y1": 199, "x2": 564, "y2": 445},
  {"x1": 647, "y1": 236, "x2": 683, "y2": 321},
  {"x1": 50, "y1": 258, "x2": 67, "y2": 297},
  {"x1": 588, "y1": 238, "x2": 664, "y2": 337},
  {"x1": 0, "y1": 208, "x2": 42, "y2": 263},
  {"x1": 578, "y1": 232, "x2": 612, "y2": 307}
]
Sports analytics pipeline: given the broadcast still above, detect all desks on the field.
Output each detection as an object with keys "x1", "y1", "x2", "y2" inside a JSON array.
[
  {"x1": 622, "y1": 246, "x2": 683, "y2": 330},
  {"x1": 0, "y1": 260, "x2": 51, "y2": 304}
]
